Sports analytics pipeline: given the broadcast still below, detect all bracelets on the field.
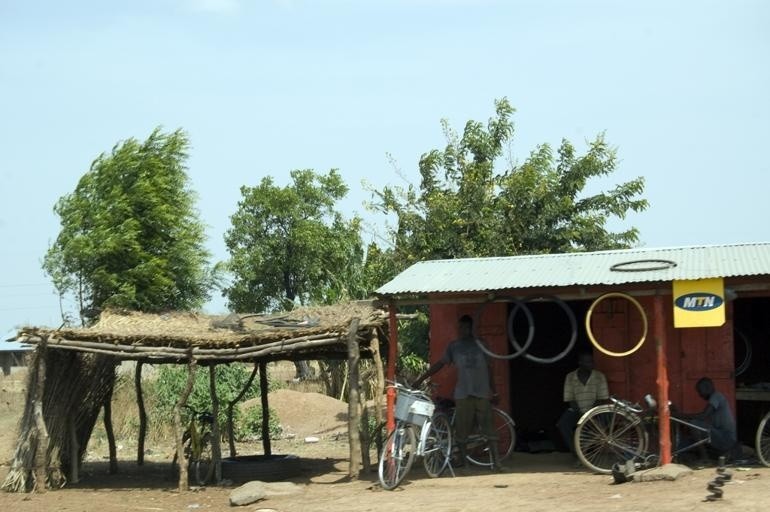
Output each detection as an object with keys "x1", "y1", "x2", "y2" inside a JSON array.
[{"x1": 493, "y1": 391, "x2": 499, "y2": 396}]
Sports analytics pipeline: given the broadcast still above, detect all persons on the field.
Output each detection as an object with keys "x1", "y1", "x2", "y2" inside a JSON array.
[
  {"x1": 556, "y1": 344, "x2": 610, "y2": 468},
  {"x1": 674, "y1": 375, "x2": 738, "y2": 466},
  {"x1": 413, "y1": 313, "x2": 503, "y2": 474}
]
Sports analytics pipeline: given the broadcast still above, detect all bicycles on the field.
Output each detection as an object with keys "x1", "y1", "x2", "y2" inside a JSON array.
[
  {"x1": 379, "y1": 378, "x2": 517, "y2": 491},
  {"x1": 573, "y1": 393, "x2": 714, "y2": 474},
  {"x1": 756, "y1": 410, "x2": 770, "y2": 470},
  {"x1": 168, "y1": 404, "x2": 219, "y2": 483}
]
